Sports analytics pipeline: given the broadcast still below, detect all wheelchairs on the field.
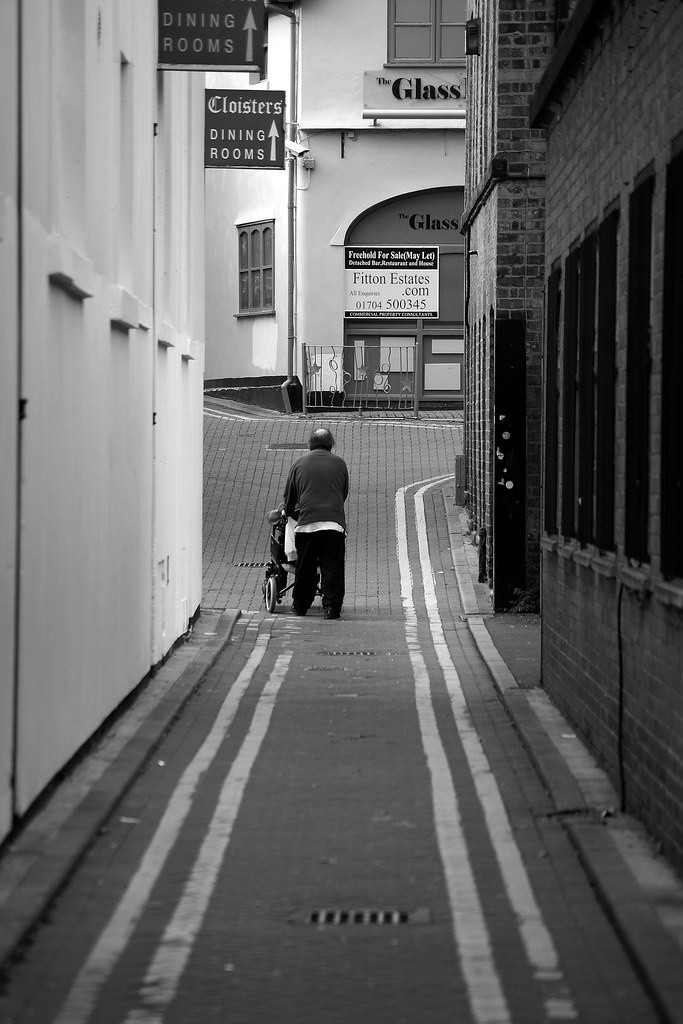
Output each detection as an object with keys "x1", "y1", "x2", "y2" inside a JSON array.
[{"x1": 261, "y1": 509, "x2": 326, "y2": 613}]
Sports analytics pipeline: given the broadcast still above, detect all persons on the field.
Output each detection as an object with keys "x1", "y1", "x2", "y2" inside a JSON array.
[
  {"x1": 262, "y1": 499, "x2": 300, "y2": 602},
  {"x1": 284, "y1": 430, "x2": 349, "y2": 621}
]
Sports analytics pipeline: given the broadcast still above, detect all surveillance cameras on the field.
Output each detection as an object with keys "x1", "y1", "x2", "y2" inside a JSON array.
[{"x1": 285, "y1": 140, "x2": 310, "y2": 158}]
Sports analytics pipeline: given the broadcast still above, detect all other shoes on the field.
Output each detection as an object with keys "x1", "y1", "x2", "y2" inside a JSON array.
[
  {"x1": 291, "y1": 604, "x2": 306, "y2": 616},
  {"x1": 324, "y1": 608, "x2": 341, "y2": 619}
]
